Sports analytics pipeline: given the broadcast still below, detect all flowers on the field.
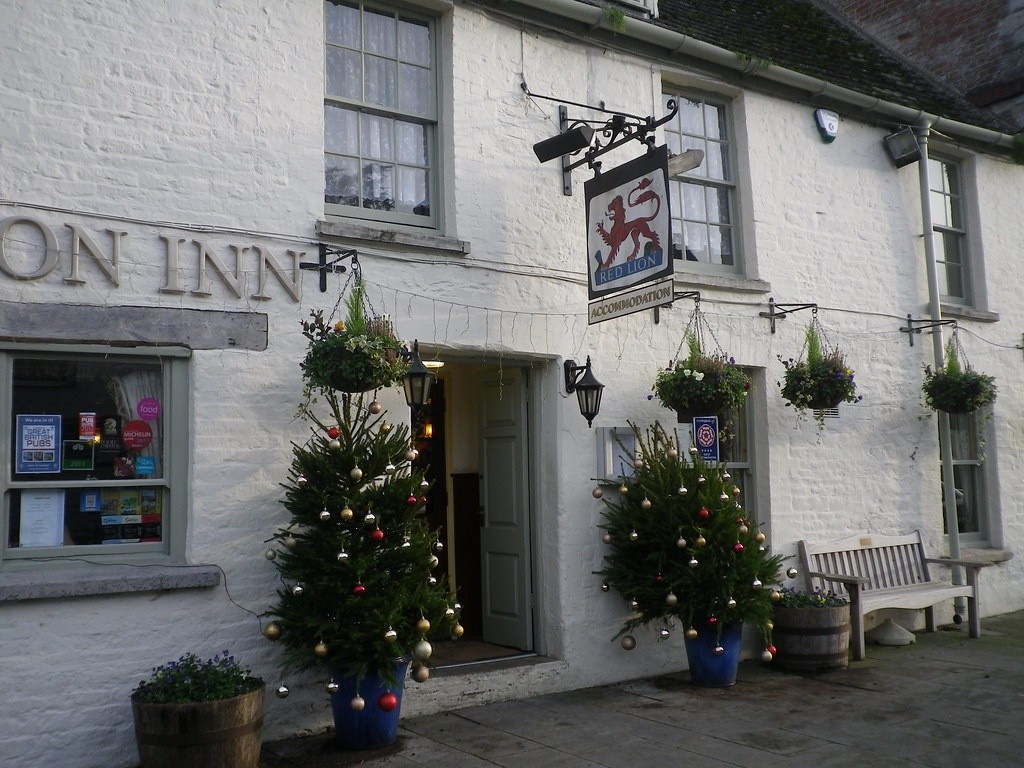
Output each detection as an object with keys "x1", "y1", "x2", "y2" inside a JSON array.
[
  {"x1": 652, "y1": 335, "x2": 749, "y2": 445},
  {"x1": 299, "y1": 311, "x2": 406, "y2": 393},
  {"x1": 919, "y1": 340, "x2": 995, "y2": 445},
  {"x1": 776, "y1": 585, "x2": 846, "y2": 612},
  {"x1": 780, "y1": 333, "x2": 857, "y2": 439},
  {"x1": 142, "y1": 652, "x2": 256, "y2": 703}
]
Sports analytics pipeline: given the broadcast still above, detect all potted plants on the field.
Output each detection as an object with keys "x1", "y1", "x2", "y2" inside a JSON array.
[
  {"x1": 260, "y1": 385, "x2": 467, "y2": 748},
  {"x1": 589, "y1": 417, "x2": 799, "y2": 691}
]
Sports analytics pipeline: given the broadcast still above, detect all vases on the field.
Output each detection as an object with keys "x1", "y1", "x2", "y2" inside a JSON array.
[
  {"x1": 779, "y1": 605, "x2": 851, "y2": 668},
  {"x1": 676, "y1": 384, "x2": 726, "y2": 425},
  {"x1": 929, "y1": 383, "x2": 983, "y2": 415},
  {"x1": 326, "y1": 346, "x2": 400, "y2": 393},
  {"x1": 132, "y1": 681, "x2": 266, "y2": 766},
  {"x1": 803, "y1": 379, "x2": 853, "y2": 411}
]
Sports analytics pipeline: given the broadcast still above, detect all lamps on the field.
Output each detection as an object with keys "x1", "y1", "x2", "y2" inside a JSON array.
[
  {"x1": 885, "y1": 127, "x2": 925, "y2": 167},
  {"x1": 532, "y1": 120, "x2": 598, "y2": 164},
  {"x1": 564, "y1": 354, "x2": 604, "y2": 428},
  {"x1": 404, "y1": 338, "x2": 439, "y2": 424},
  {"x1": 646, "y1": 145, "x2": 705, "y2": 178}
]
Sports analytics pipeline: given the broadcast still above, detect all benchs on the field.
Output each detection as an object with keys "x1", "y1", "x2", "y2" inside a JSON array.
[{"x1": 798, "y1": 529, "x2": 991, "y2": 662}]
